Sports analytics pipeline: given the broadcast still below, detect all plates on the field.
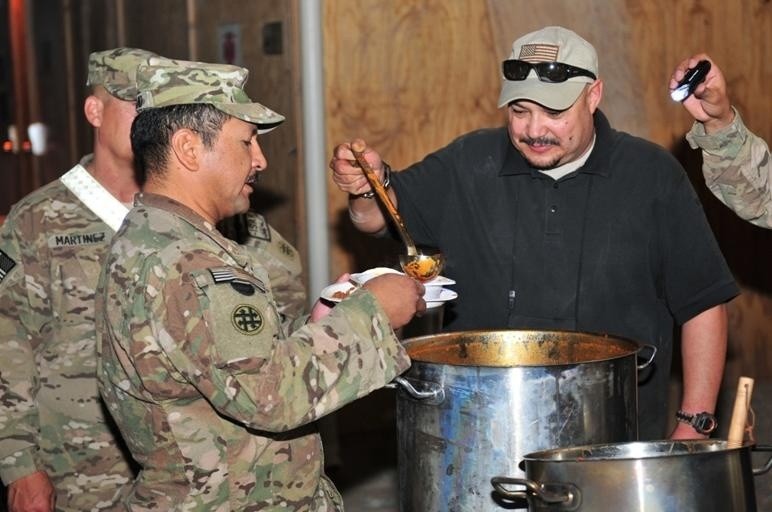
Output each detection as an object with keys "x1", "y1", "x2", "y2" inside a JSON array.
[{"x1": 319, "y1": 264, "x2": 459, "y2": 311}]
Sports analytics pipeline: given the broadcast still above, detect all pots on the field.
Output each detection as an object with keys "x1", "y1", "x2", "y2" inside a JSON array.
[
  {"x1": 376, "y1": 324, "x2": 660, "y2": 512},
  {"x1": 489, "y1": 439, "x2": 772, "y2": 512}
]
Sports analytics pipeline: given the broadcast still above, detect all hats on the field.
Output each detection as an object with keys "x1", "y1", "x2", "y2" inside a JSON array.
[
  {"x1": 137, "y1": 64, "x2": 285, "y2": 135},
  {"x1": 497, "y1": 25, "x2": 599, "y2": 110},
  {"x1": 86, "y1": 47, "x2": 153, "y2": 102}
]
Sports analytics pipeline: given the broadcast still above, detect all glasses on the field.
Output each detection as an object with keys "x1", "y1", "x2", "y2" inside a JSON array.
[{"x1": 503, "y1": 59, "x2": 597, "y2": 83}]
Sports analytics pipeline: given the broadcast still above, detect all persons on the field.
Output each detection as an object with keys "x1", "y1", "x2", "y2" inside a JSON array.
[
  {"x1": 1, "y1": 48, "x2": 309, "y2": 510},
  {"x1": 329, "y1": 21, "x2": 744, "y2": 442},
  {"x1": 667, "y1": 49, "x2": 772, "y2": 232},
  {"x1": 97, "y1": 53, "x2": 428, "y2": 510}
]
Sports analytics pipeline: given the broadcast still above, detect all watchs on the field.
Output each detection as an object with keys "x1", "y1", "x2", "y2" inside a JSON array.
[
  {"x1": 359, "y1": 160, "x2": 392, "y2": 199},
  {"x1": 676, "y1": 410, "x2": 719, "y2": 435}
]
